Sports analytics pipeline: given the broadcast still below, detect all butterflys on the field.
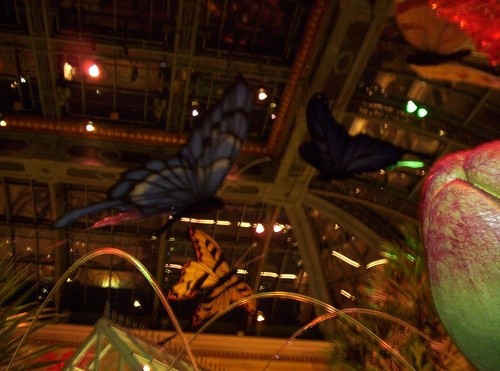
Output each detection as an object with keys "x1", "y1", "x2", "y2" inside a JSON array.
[
  {"x1": 154, "y1": 227, "x2": 258, "y2": 349},
  {"x1": 298, "y1": 93, "x2": 407, "y2": 180},
  {"x1": 50, "y1": 75, "x2": 256, "y2": 236},
  {"x1": 393, "y1": 1, "x2": 476, "y2": 65}
]
